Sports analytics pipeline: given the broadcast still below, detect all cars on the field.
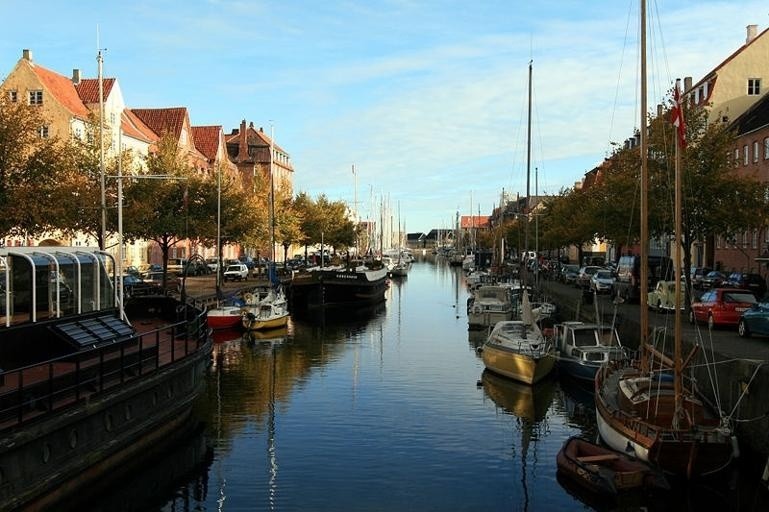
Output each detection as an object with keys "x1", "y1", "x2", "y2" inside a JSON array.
[
  {"x1": 102, "y1": 249, "x2": 344, "y2": 301},
  {"x1": 619, "y1": 243, "x2": 768, "y2": 346}
]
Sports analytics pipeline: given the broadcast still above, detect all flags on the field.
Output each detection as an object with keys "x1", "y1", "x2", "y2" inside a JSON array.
[{"x1": 671, "y1": 84, "x2": 688, "y2": 150}]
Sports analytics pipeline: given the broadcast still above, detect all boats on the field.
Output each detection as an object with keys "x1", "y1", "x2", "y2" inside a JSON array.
[
  {"x1": 550, "y1": 469, "x2": 630, "y2": 512},
  {"x1": 211, "y1": 329, "x2": 244, "y2": 347},
  {"x1": 249, "y1": 320, "x2": 299, "y2": 341},
  {"x1": 208, "y1": 293, "x2": 243, "y2": 330},
  {"x1": 241, "y1": 284, "x2": 289, "y2": 334},
  {"x1": 550, "y1": 435, "x2": 656, "y2": 497}
]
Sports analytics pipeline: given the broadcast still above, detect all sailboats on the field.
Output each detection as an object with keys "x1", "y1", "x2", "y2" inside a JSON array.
[
  {"x1": 472, "y1": 367, "x2": 556, "y2": 511},
  {"x1": 315, "y1": 160, "x2": 418, "y2": 307},
  {"x1": 593, "y1": 1, "x2": 741, "y2": 482}
]
